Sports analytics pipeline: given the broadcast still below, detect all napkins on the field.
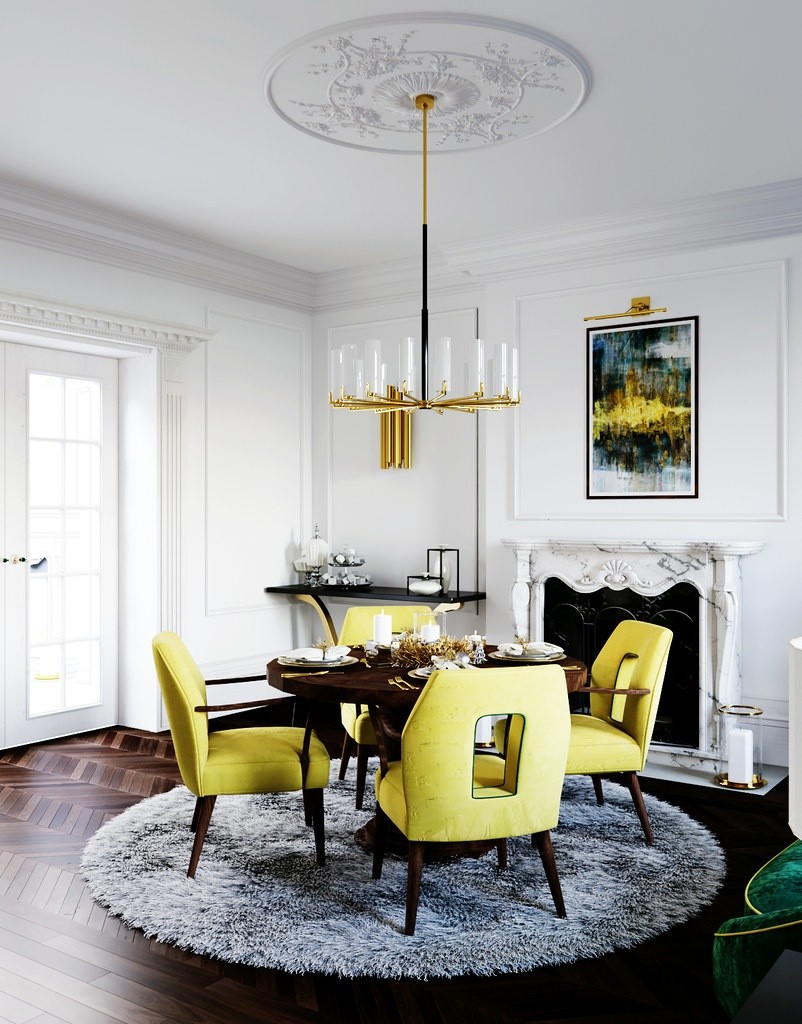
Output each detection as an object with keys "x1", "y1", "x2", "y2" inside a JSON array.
[
  {"x1": 432, "y1": 655, "x2": 467, "y2": 670},
  {"x1": 497, "y1": 635, "x2": 564, "y2": 656},
  {"x1": 280, "y1": 641, "x2": 351, "y2": 660}
]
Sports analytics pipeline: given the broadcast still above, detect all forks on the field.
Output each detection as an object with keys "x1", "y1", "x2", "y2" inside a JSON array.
[{"x1": 388, "y1": 676, "x2": 420, "y2": 690}]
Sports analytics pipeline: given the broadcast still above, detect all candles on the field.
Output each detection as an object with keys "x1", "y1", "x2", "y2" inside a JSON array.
[
  {"x1": 373, "y1": 608, "x2": 392, "y2": 644},
  {"x1": 728, "y1": 727, "x2": 753, "y2": 783},
  {"x1": 421, "y1": 619, "x2": 440, "y2": 642},
  {"x1": 470, "y1": 630, "x2": 481, "y2": 642}
]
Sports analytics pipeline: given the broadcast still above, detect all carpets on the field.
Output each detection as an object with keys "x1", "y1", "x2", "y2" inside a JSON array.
[{"x1": 82, "y1": 758, "x2": 725, "y2": 977}]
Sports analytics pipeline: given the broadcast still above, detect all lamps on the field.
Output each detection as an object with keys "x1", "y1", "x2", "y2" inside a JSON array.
[
  {"x1": 306, "y1": 522, "x2": 327, "y2": 587},
  {"x1": 380, "y1": 385, "x2": 412, "y2": 468},
  {"x1": 329, "y1": 95, "x2": 520, "y2": 414}
]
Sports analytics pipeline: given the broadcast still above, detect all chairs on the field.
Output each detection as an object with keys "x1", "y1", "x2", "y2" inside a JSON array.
[{"x1": 152, "y1": 607, "x2": 673, "y2": 936}]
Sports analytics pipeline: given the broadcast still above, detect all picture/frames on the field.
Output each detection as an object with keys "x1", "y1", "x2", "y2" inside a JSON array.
[{"x1": 585, "y1": 315, "x2": 699, "y2": 499}]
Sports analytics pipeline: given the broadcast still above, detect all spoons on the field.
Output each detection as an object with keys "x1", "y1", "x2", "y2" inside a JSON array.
[{"x1": 360, "y1": 658, "x2": 371, "y2": 668}]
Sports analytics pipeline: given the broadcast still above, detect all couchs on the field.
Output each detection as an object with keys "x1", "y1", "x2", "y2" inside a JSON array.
[{"x1": 713, "y1": 839, "x2": 802, "y2": 1018}]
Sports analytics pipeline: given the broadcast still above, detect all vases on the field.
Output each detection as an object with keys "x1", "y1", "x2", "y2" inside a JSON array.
[{"x1": 409, "y1": 544, "x2": 452, "y2": 596}]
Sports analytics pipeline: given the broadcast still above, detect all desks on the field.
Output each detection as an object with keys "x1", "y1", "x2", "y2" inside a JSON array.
[{"x1": 264, "y1": 584, "x2": 486, "y2": 646}]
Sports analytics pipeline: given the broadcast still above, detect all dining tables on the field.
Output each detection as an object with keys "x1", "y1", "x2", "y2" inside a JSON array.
[{"x1": 267, "y1": 637, "x2": 587, "y2": 863}]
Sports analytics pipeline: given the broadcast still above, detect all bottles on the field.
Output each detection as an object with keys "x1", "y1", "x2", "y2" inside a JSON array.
[
  {"x1": 470, "y1": 630, "x2": 481, "y2": 650},
  {"x1": 434, "y1": 544, "x2": 451, "y2": 594},
  {"x1": 481, "y1": 635, "x2": 487, "y2": 649},
  {"x1": 409, "y1": 572, "x2": 443, "y2": 595}
]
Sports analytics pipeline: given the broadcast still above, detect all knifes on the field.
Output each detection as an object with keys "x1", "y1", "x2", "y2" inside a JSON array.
[{"x1": 281, "y1": 671, "x2": 329, "y2": 678}]
